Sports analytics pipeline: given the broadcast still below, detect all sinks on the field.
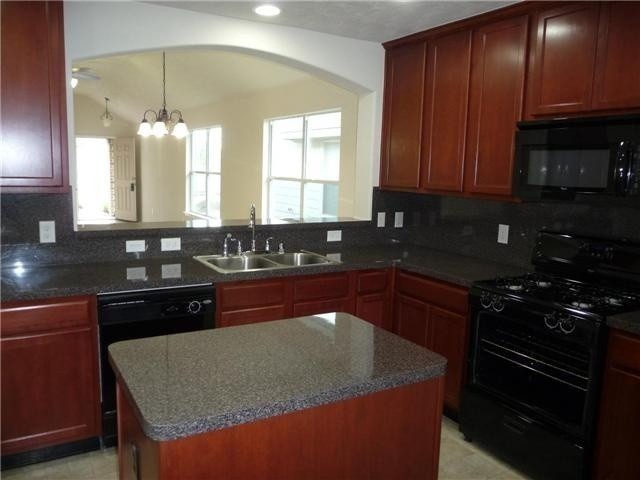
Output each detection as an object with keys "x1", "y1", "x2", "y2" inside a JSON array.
[
  {"x1": 192, "y1": 251, "x2": 276, "y2": 275},
  {"x1": 264, "y1": 249, "x2": 338, "y2": 269}
]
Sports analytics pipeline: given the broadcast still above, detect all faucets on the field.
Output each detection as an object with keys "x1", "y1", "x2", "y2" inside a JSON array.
[
  {"x1": 247, "y1": 206, "x2": 257, "y2": 253},
  {"x1": 222, "y1": 236, "x2": 243, "y2": 258}
]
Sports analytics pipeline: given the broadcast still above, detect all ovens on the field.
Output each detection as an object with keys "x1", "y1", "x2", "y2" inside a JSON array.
[{"x1": 460, "y1": 286, "x2": 604, "y2": 479}]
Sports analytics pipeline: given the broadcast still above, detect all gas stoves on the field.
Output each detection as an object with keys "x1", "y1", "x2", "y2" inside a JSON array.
[{"x1": 476, "y1": 230, "x2": 640, "y2": 316}]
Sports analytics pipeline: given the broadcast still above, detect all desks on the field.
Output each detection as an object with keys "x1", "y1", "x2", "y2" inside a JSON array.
[{"x1": 109, "y1": 311, "x2": 448, "y2": 480}]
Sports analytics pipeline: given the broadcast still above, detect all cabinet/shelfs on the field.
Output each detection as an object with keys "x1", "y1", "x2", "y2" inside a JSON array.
[
  {"x1": 420, "y1": 17, "x2": 473, "y2": 198},
  {"x1": 0, "y1": 294, "x2": 103, "y2": 471},
  {"x1": 354, "y1": 268, "x2": 396, "y2": 333},
  {"x1": 379, "y1": 30, "x2": 427, "y2": 193},
  {"x1": 0, "y1": 0, "x2": 70, "y2": 193},
  {"x1": 394, "y1": 268, "x2": 471, "y2": 411},
  {"x1": 466, "y1": 1, "x2": 530, "y2": 203},
  {"x1": 594, "y1": 328, "x2": 640, "y2": 480},
  {"x1": 523, "y1": 0, "x2": 640, "y2": 119},
  {"x1": 215, "y1": 271, "x2": 354, "y2": 328}
]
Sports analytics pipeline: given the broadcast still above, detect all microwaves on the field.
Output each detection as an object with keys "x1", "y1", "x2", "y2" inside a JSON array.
[{"x1": 509, "y1": 114, "x2": 640, "y2": 206}]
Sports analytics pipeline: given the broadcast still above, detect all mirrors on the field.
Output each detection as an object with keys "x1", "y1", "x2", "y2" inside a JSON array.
[{"x1": 67, "y1": 47, "x2": 360, "y2": 232}]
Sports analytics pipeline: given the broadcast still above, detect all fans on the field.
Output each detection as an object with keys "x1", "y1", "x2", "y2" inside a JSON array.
[{"x1": 72, "y1": 66, "x2": 100, "y2": 81}]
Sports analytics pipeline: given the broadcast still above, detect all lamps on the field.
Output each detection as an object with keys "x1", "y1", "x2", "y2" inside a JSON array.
[
  {"x1": 137, "y1": 50, "x2": 190, "y2": 140},
  {"x1": 99, "y1": 97, "x2": 113, "y2": 128}
]
